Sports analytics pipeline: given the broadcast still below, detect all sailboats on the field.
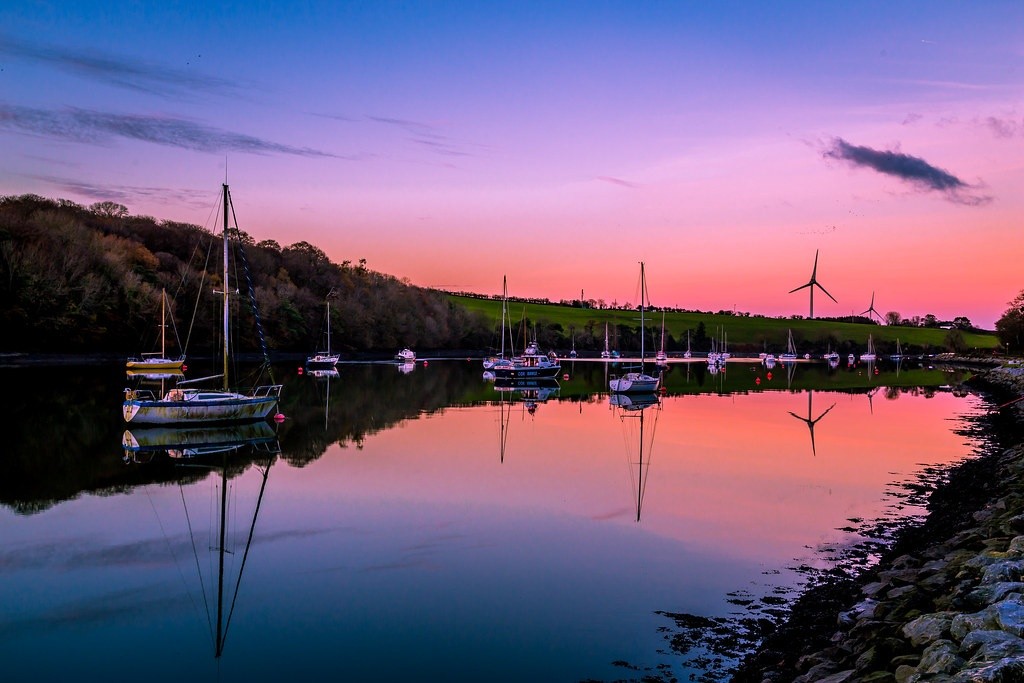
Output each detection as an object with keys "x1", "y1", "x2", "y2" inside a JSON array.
[
  {"x1": 493, "y1": 378, "x2": 562, "y2": 466},
  {"x1": 609, "y1": 261, "x2": 660, "y2": 393},
  {"x1": 665, "y1": 363, "x2": 913, "y2": 393},
  {"x1": 123, "y1": 157, "x2": 284, "y2": 423},
  {"x1": 305, "y1": 287, "x2": 341, "y2": 366},
  {"x1": 304, "y1": 366, "x2": 340, "y2": 430},
  {"x1": 608, "y1": 393, "x2": 661, "y2": 522},
  {"x1": 565, "y1": 312, "x2": 906, "y2": 363},
  {"x1": 483, "y1": 275, "x2": 562, "y2": 379},
  {"x1": 119, "y1": 424, "x2": 281, "y2": 680}
]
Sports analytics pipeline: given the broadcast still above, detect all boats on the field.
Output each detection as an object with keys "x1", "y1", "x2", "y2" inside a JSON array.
[
  {"x1": 394, "y1": 349, "x2": 416, "y2": 363},
  {"x1": 396, "y1": 363, "x2": 416, "y2": 375}
]
